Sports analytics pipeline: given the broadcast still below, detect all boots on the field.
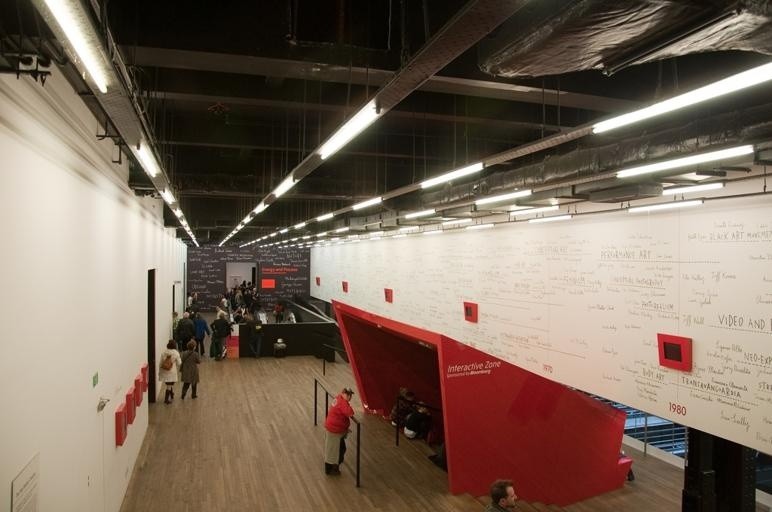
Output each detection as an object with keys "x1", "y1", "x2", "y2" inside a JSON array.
[{"x1": 164, "y1": 387, "x2": 174, "y2": 404}]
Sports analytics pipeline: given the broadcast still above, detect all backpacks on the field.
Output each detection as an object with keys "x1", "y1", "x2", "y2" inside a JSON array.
[{"x1": 162, "y1": 355, "x2": 176, "y2": 370}]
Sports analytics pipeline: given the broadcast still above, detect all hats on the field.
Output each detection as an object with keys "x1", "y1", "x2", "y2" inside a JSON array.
[{"x1": 344, "y1": 388, "x2": 355, "y2": 394}]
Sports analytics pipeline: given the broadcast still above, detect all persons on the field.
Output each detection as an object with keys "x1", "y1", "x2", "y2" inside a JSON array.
[
  {"x1": 483, "y1": 479, "x2": 517, "y2": 512},
  {"x1": 179, "y1": 339, "x2": 201, "y2": 401},
  {"x1": 323, "y1": 386, "x2": 355, "y2": 476},
  {"x1": 403, "y1": 402, "x2": 433, "y2": 440},
  {"x1": 158, "y1": 338, "x2": 182, "y2": 405},
  {"x1": 175, "y1": 279, "x2": 285, "y2": 361},
  {"x1": 391, "y1": 391, "x2": 418, "y2": 427}
]
